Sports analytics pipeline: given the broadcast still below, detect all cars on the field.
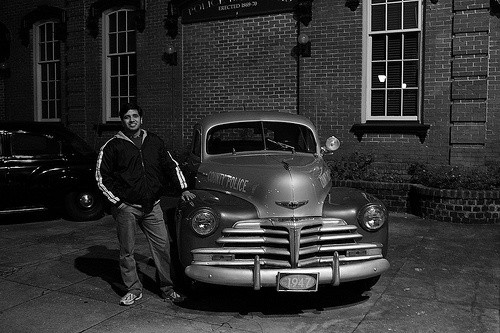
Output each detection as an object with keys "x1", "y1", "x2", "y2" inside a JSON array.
[
  {"x1": 0, "y1": 119, "x2": 112, "y2": 222},
  {"x1": 173, "y1": 110, "x2": 390, "y2": 290}
]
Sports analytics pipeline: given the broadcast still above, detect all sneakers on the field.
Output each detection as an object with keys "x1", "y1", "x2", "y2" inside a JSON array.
[
  {"x1": 120, "y1": 291, "x2": 143, "y2": 306},
  {"x1": 162, "y1": 288, "x2": 185, "y2": 302}
]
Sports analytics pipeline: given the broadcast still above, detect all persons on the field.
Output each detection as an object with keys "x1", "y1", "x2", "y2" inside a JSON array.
[{"x1": 92, "y1": 100, "x2": 198, "y2": 305}]
